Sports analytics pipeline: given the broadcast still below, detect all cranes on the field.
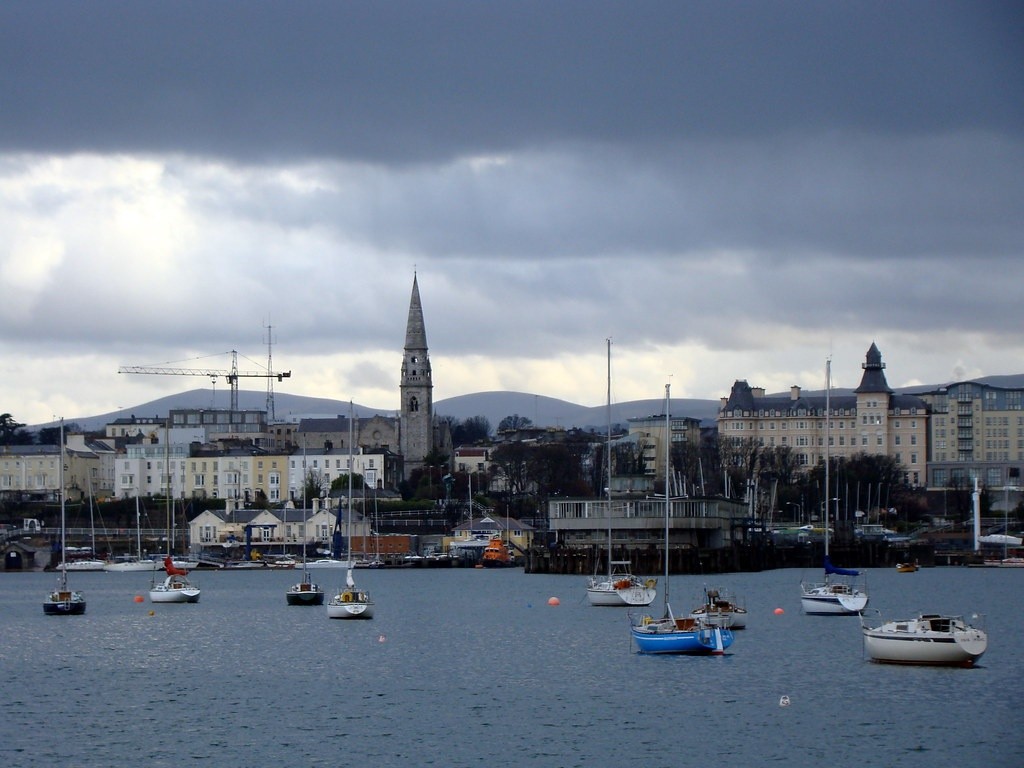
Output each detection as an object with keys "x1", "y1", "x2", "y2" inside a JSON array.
[{"x1": 117, "y1": 351, "x2": 292, "y2": 433}]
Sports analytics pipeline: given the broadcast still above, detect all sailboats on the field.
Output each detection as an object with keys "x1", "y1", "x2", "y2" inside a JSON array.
[
  {"x1": 584, "y1": 336, "x2": 657, "y2": 607},
  {"x1": 286, "y1": 432, "x2": 324, "y2": 607},
  {"x1": 148, "y1": 419, "x2": 201, "y2": 604},
  {"x1": 157, "y1": 472, "x2": 200, "y2": 570},
  {"x1": 103, "y1": 487, "x2": 163, "y2": 572},
  {"x1": 796, "y1": 352, "x2": 870, "y2": 615},
  {"x1": 626, "y1": 383, "x2": 736, "y2": 657},
  {"x1": 327, "y1": 399, "x2": 377, "y2": 618},
  {"x1": 41, "y1": 415, "x2": 87, "y2": 616},
  {"x1": 56, "y1": 462, "x2": 116, "y2": 572},
  {"x1": 980, "y1": 467, "x2": 1024, "y2": 568}
]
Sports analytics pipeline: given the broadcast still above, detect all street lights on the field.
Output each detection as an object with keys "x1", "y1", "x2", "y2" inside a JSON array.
[
  {"x1": 786, "y1": 502, "x2": 800, "y2": 529},
  {"x1": 819, "y1": 498, "x2": 841, "y2": 534}
]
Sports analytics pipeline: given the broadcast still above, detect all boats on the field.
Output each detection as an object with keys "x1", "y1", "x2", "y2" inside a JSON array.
[
  {"x1": 857, "y1": 610, "x2": 991, "y2": 667},
  {"x1": 896, "y1": 563, "x2": 917, "y2": 572},
  {"x1": 689, "y1": 588, "x2": 748, "y2": 630},
  {"x1": 294, "y1": 559, "x2": 355, "y2": 569}
]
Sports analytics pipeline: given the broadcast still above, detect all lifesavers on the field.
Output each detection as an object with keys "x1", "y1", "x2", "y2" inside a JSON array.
[{"x1": 342, "y1": 592, "x2": 352, "y2": 602}]
